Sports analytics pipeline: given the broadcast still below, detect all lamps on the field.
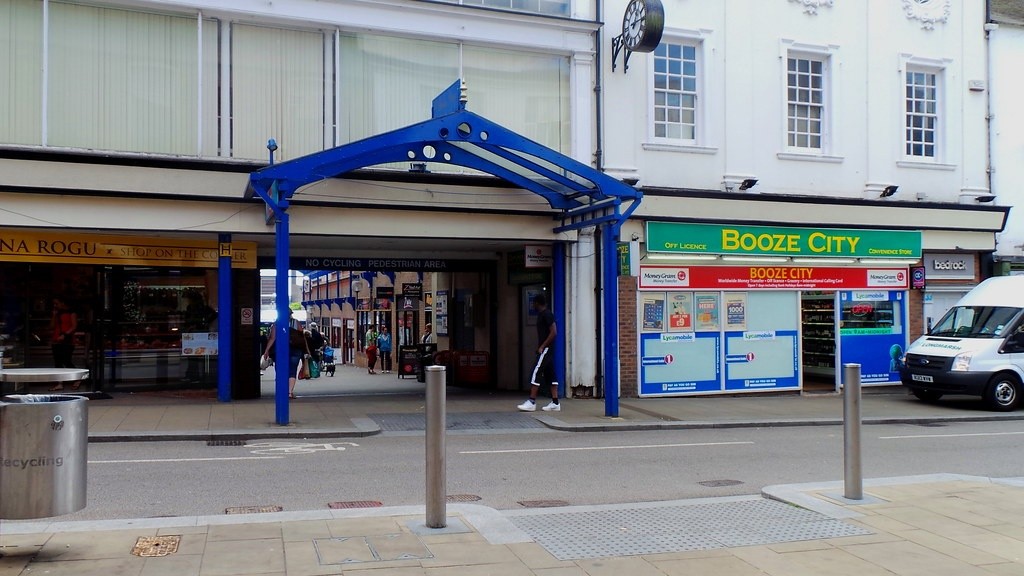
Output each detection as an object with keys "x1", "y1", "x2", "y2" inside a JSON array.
[
  {"x1": 738, "y1": 179, "x2": 760, "y2": 190},
  {"x1": 879, "y1": 184, "x2": 904, "y2": 199},
  {"x1": 974, "y1": 194, "x2": 997, "y2": 204},
  {"x1": 618, "y1": 177, "x2": 643, "y2": 188},
  {"x1": 350, "y1": 275, "x2": 365, "y2": 297},
  {"x1": 265, "y1": 138, "x2": 280, "y2": 151}
]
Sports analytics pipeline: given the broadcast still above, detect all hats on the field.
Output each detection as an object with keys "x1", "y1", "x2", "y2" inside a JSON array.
[{"x1": 309, "y1": 322, "x2": 317, "y2": 329}]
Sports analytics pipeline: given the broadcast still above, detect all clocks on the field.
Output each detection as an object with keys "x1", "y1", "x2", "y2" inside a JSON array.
[{"x1": 618, "y1": 0, "x2": 667, "y2": 53}]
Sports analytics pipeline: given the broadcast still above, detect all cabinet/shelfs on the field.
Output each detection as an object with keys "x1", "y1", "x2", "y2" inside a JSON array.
[{"x1": 801, "y1": 288, "x2": 896, "y2": 376}]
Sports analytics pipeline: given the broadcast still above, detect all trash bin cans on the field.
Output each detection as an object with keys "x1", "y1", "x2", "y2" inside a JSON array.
[
  {"x1": 0, "y1": 367, "x2": 90, "y2": 520},
  {"x1": 417, "y1": 344, "x2": 437, "y2": 383}
]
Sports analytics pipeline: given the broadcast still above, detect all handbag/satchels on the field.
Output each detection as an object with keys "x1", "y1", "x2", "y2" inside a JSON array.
[{"x1": 298, "y1": 356, "x2": 320, "y2": 380}]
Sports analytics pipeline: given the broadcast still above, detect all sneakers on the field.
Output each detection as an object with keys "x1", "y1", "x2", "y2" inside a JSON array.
[
  {"x1": 517, "y1": 399, "x2": 536, "y2": 411},
  {"x1": 541, "y1": 401, "x2": 561, "y2": 412}
]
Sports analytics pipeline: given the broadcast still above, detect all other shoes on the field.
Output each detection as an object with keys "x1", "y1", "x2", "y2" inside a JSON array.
[
  {"x1": 386, "y1": 371, "x2": 389, "y2": 373},
  {"x1": 382, "y1": 371, "x2": 384, "y2": 373},
  {"x1": 289, "y1": 393, "x2": 296, "y2": 399},
  {"x1": 368, "y1": 369, "x2": 376, "y2": 374}
]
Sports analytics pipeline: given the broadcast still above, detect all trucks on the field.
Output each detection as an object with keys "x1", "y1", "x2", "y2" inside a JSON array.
[{"x1": 895, "y1": 275, "x2": 1024, "y2": 412}]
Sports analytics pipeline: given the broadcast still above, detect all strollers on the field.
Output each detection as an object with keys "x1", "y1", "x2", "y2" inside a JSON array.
[{"x1": 319, "y1": 346, "x2": 336, "y2": 378}]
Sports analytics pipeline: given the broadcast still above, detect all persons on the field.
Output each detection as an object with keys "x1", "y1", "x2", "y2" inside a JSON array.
[
  {"x1": 517, "y1": 293, "x2": 561, "y2": 411},
  {"x1": 421, "y1": 323, "x2": 433, "y2": 344},
  {"x1": 48, "y1": 295, "x2": 83, "y2": 392},
  {"x1": 300, "y1": 322, "x2": 335, "y2": 376},
  {"x1": 264, "y1": 308, "x2": 312, "y2": 398},
  {"x1": 178, "y1": 293, "x2": 218, "y2": 383},
  {"x1": 364, "y1": 324, "x2": 378, "y2": 374},
  {"x1": 261, "y1": 329, "x2": 267, "y2": 355},
  {"x1": 378, "y1": 326, "x2": 393, "y2": 372}
]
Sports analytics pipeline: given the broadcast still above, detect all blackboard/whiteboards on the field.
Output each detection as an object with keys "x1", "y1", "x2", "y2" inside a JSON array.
[{"x1": 398, "y1": 345, "x2": 418, "y2": 375}]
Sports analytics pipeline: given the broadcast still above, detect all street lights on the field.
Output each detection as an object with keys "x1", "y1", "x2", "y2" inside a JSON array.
[
  {"x1": 309, "y1": 306, "x2": 315, "y2": 323},
  {"x1": 351, "y1": 278, "x2": 362, "y2": 352}
]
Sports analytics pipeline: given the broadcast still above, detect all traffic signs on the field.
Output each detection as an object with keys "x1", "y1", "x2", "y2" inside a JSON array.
[{"x1": 399, "y1": 344, "x2": 423, "y2": 378}]
